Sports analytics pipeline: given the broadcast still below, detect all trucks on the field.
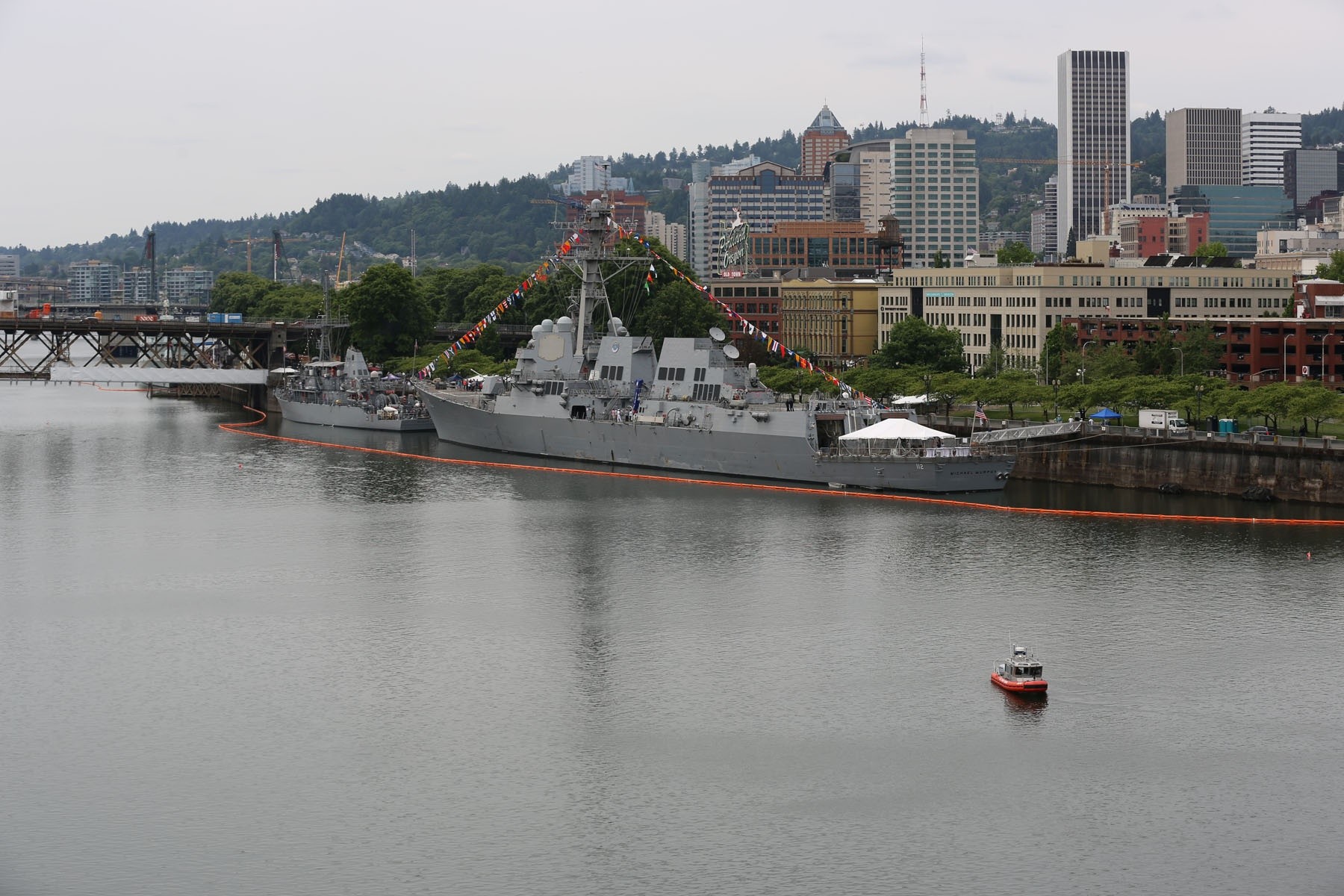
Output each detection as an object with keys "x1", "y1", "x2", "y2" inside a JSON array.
[{"x1": 1138, "y1": 407, "x2": 1188, "y2": 436}]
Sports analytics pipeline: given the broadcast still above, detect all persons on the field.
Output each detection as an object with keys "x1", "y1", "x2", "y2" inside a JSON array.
[
  {"x1": 732, "y1": 393, "x2": 746, "y2": 410},
  {"x1": 277, "y1": 363, "x2": 430, "y2": 420},
  {"x1": 611, "y1": 408, "x2": 640, "y2": 423},
  {"x1": 1079, "y1": 417, "x2": 1093, "y2": 431},
  {"x1": 789, "y1": 398, "x2": 795, "y2": 411},
  {"x1": 786, "y1": 399, "x2": 790, "y2": 412},
  {"x1": 456, "y1": 377, "x2": 483, "y2": 392},
  {"x1": 590, "y1": 407, "x2": 597, "y2": 420},
  {"x1": 1290, "y1": 422, "x2": 1306, "y2": 442},
  {"x1": 1068, "y1": 416, "x2": 1074, "y2": 423},
  {"x1": 1055, "y1": 414, "x2": 1064, "y2": 424}
]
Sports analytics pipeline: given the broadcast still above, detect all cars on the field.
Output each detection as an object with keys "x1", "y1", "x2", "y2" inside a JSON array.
[{"x1": 27, "y1": 310, "x2": 98, "y2": 322}]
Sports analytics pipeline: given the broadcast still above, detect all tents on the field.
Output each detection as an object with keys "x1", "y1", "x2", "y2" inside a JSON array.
[{"x1": 1090, "y1": 408, "x2": 1124, "y2": 426}]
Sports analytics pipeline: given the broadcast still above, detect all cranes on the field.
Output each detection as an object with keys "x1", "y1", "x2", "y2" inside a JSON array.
[
  {"x1": 968, "y1": 151, "x2": 1145, "y2": 235},
  {"x1": 529, "y1": 188, "x2": 650, "y2": 252},
  {"x1": 227, "y1": 235, "x2": 305, "y2": 278}
]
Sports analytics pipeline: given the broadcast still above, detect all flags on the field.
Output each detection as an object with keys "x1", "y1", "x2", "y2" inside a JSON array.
[
  {"x1": 416, "y1": 228, "x2": 588, "y2": 382},
  {"x1": 816, "y1": 365, "x2": 892, "y2": 413},
  {"x1": 643, "y1": 263, "x2": 657, "y2": 296},
  {"x1": 663, "y1": 260, "x2": 814, "y2": 377},
  {"x1": 975, "y1": 406, "x2": 988, "y2": 423},
  {"x1": 605, "y1": 216, "x2": 662, "y2": 261}
]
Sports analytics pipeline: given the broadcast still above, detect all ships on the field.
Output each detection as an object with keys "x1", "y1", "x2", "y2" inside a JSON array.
[{"x1": 408, "y1": 161, "x2": 1020, "y2": 495}]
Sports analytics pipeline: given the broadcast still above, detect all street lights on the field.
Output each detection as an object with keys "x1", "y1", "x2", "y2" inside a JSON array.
[
  {"x1": 796, "y1": 370, "x2": 803, "y2": 404},
  {"x1": 447, "y1": 355, "x2": 454, "y2": 378},
  {"x1": 923, "y1": 374, "x2": 932, "y2": 415},
  {"x1": 985, "y1": 342, "x2": 997, "y2": 380},
  {"x1": 504, "y1": 358, "x2": 510, "y2": 390},
  {"x1": 1081, "y1": 341, "x2": 1096, "y2": 386},
  {"x1": 1195, "y1": 384, "x2": 1204, "y2": 430},
  {"x1": 1171, "y1": 348, "x2": 1185, "y2": 376},
  {"x1": 1283, "y1": 333, "x2": 1295, "y2": 380},
  {"x1": 1051, "y1": 379, "x2": 1061, "y2": 418},
  {"x1": 1321, "y1": 333, "x2": 1334, "y2": 384},
  {"x1": 1035, "y1": 333, "x2": 1049, "y2": 386}
]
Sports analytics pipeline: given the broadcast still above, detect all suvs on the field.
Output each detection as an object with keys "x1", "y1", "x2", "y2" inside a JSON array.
[{"x1": 1240, "y1": 425, "x2": 1279, "y2": 442}]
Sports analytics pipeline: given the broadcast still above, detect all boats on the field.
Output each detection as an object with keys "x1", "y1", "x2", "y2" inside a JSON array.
[
  {"x1": 271, "y1": 269, "x2": 437, "y2": 433},
  {"x1": 989, "y1": 630, "x2": 1048, "y2": 693}
]
